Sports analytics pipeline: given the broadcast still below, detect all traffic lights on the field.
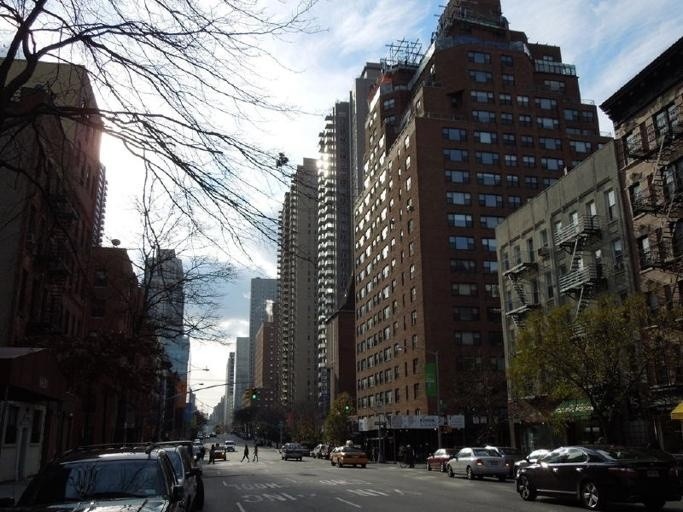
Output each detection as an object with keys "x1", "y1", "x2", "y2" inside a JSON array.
[
  {"x1": 344, "y1": 400, "x2": 352, "y2": 413},
  {"x1": 250, "y1": 387, "x2": 258, "y2": 400}
]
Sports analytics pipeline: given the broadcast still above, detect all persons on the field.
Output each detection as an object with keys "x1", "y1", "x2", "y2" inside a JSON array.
[
  {"x1": 241, "y1": 444, "x2": 249, "y2": 463},
  {"x1": 398, "y1": 444, "x2": 415, "y2": 468},
  {"x1": 199, "y1": 442, "x2": 205, "y2": 464},
  {"x1": 371, "y1": 444, "x2": 379, "y2": 461},
  {"x1": 208, "y1": 447, "x2": 215, "y2": 464},
  {"x1": 252, "y1": 444, "x2": 258, "y2": 462}
]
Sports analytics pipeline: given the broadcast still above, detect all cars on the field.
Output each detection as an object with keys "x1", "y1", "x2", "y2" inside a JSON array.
[
  {"x1": 426, "y1": 444, "x2": 683, "y2": 511},
  {"x1": 11, "y1": 439, "x2": 207, "y2": 512},
  {"x1": 197, "y1": 430, "x2": 217, "y2": 440},
  {"x1": 214, "y1": 440, "x2": 236, "y2": 461},
  {"x1": 280, "y1": 442, "x2": 369, "y2": 468}
]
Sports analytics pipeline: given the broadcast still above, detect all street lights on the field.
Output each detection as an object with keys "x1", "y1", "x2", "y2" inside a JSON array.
[
  {"x1": 395, "y1": 344, "x2": 442, "y2": 449},
  {"x1": 162, "y1": 368, "x2": 209, "y2": 421}
]
